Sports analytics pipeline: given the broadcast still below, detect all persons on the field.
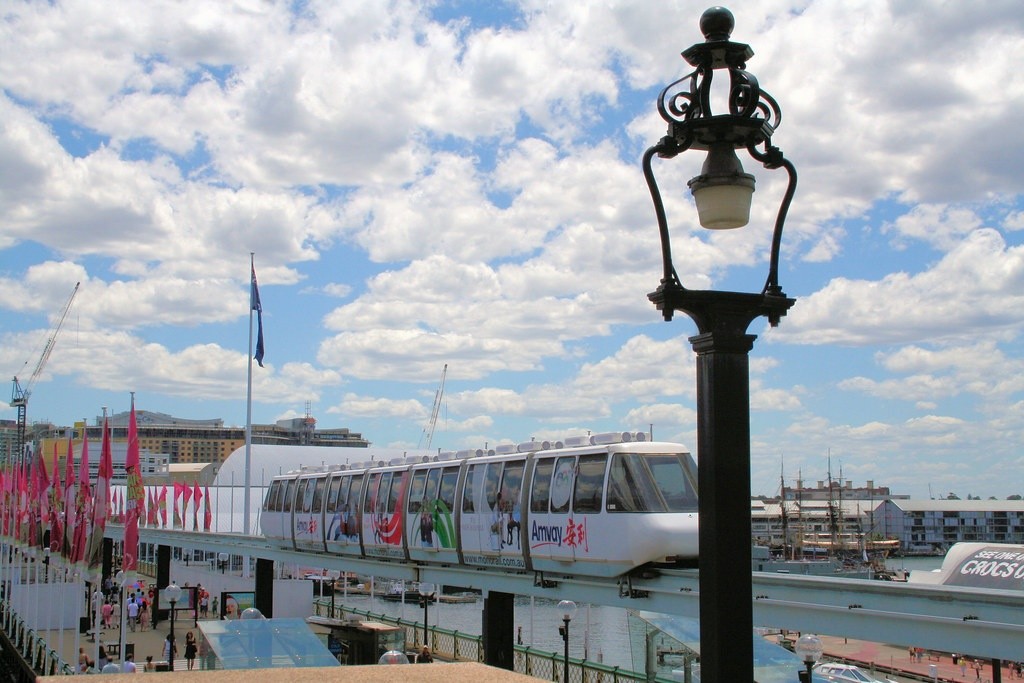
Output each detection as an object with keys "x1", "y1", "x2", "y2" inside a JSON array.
[
  {"x1": 908, "y1": 646, "x2": 924, "y2": 663},
  {"x1": 226, "y1": 595, "x2": 239, "y2": 614},
  {"x1": 374, "y1": 514, "x2": 381, "y2": 544},
  {"x1": 92, "y1": 554, "x2": 150, "y2": 632},
  {"x1": 183, "y1": 631, "x2": 197, "y2": 670},
  {"x1": 196, "y1": 584, "x2": 219, "y2": 619},
  {"x1": 1008, "y1": 662, "x2": 1024, "y2": 680},
  {"x1": 490, "y1": 492, "x2": 520, "y2": 551},
  {"x1": 333, "y1": 504, "x2": 359, "y2": 541},
  {"x1": 312, "y1": 579, "x2": 332, "y2": 596},
  {"x1": 975, "y1": 659, "x2": 980, "y2": 679},
  {"x1": 143, "y1": 655, "x2": 156, "y2": 672},
  {"x1": 78, "y1": 645, "x2": 137, "y2": 674},
  {"x1": 420, "y1": 511, "x2": 433, "y2": 547},
  {"x1": 416, "y1": 645, "x2": 433, "y2": 663},
  {"x1": 959, "y1": 658, "x2": 967, "y2": 676},
  {"x1": 162, "y1": 634, "x2": 179, "y2": 663}
]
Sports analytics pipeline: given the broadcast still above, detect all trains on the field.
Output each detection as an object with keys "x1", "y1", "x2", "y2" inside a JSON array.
[{"x1": 261, "y1": 432, "x2": 699, "y2": 577}]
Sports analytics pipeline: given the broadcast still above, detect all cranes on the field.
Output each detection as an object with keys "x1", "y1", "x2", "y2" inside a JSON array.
[
  {"x1": 418, "y1": 362, "x2": 448, "y2": 450},
  {"x1": 9, "y1": 281, "x2": 80, "y2": 469}
]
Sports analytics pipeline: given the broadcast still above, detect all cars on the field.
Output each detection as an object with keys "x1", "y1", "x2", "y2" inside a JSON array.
[{"x1": 815, "y1": 664, "x2": 881, "y2": 683}]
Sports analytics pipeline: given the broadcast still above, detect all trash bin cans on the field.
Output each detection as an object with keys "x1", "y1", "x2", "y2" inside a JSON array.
[
  {"x1": 80, "y1": 617, "x2": 91, "y2": 633},
  {"x1": 115, "y1": 569, "x2": 121, "y2": 577},
  {"x1": 125, "y1": 643, "x2": 135, "y2": 661}
]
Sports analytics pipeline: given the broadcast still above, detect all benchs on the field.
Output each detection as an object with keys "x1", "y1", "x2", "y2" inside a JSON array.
[
  {"x1": 104, "y1": 640, "x2": 119, "y2": 655},
  {"x1": 86, "y1": 630, "x2": 105, "y2": 642}
]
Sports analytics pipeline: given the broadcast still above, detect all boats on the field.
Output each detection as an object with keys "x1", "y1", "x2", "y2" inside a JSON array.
[{"x1": 333, "y1": 578, "x2": 477, "y2": 603}]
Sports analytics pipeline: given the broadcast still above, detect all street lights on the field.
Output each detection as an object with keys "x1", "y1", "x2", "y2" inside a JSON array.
[
  {"x1": 219, "y1": 553, "x2": 229, "y2": 574},
  {"x1": 640, "y1": 6, "x2": 797, "y2": 683},
  {"x1": 163, "y1": 584, "x2": 181, "y2": 672},
  {"x1": 184, "y1": 548, "x2": 192, "y2": 566},
  {"x1": 116, "y1": 571, "x2": 123, "y2": 659},
  {"x1": 44, "y1": 547, "x2": 51, "y2": 583},
  {"x1": 557, "y1": 600, "x2": 577, "y2": 683},
  {"x1": 419, "y1": 583, "x2": 435, "y2": 645}
]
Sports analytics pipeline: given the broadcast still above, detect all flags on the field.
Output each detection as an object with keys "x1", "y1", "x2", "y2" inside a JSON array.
[
  {"x1": 0, "y1": 398, "x2": 212, "y2": 587},
  {"x1": 251, "y1": 268, "x2": 265, "y2": 368}
]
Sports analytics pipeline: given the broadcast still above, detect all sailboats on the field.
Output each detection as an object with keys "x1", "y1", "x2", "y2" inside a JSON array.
[{"x1": 753, "y1": 447, "x2": 910, "y2": 583}]
